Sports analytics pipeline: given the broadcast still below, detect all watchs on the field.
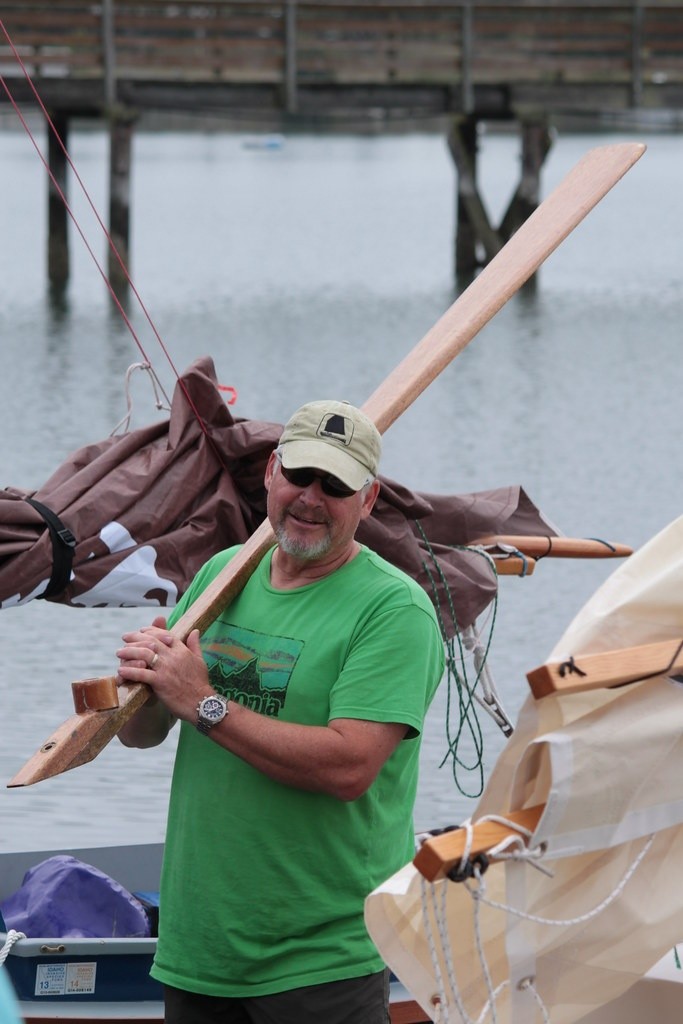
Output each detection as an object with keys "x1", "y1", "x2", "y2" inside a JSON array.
[{"x1": 195, "y1": 691, "x2": 231, "y2": 737}]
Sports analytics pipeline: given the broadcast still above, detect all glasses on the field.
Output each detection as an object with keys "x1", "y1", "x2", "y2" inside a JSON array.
[{"x1": 276, "y1": 454, "x2": 370, "y2": 499}]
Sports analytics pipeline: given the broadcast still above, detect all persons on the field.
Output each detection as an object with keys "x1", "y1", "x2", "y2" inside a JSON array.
[{"x1": 115, "y1": 398, "x2": 444, "y2": 1024}]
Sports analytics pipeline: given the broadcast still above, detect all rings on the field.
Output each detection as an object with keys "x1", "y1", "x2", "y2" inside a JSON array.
[{"x1": 147, "y1": 653, "x2": 159, "y2": 667}]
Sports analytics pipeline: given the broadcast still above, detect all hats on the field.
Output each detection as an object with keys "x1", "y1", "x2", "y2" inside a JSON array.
[{"x1": 274, "y1": 396, "x2": 384, "y2": 490}]
